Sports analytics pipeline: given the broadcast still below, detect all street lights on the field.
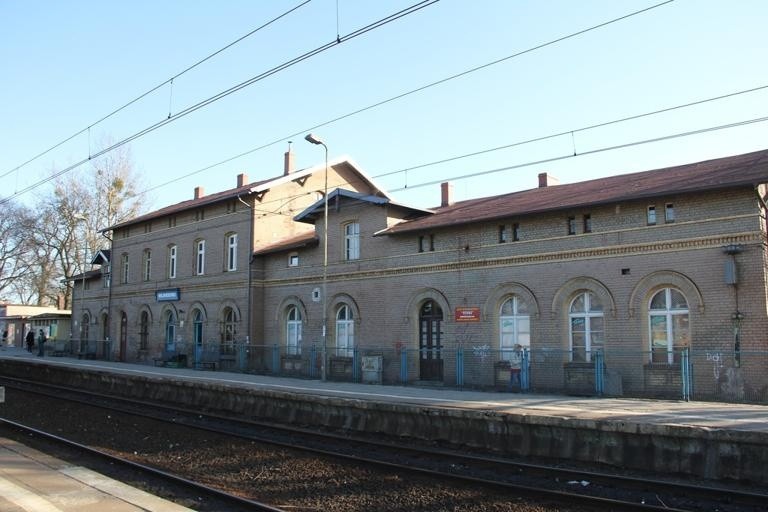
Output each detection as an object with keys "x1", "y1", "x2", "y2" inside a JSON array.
[
  {"x1": 303, "y1": 133, "x2": 328, "y2": 383},
  {"x1": 70, "y1": 213, "x2": 89, "y2": 355}
]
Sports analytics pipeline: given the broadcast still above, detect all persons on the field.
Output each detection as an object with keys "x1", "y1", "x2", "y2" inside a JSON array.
[
  {"x1": 25, "y1": 329, "x2": 35, "y2": 354},
  {"x1": 36, "y1": 329, "x2": 46, "y2": 357}
]
